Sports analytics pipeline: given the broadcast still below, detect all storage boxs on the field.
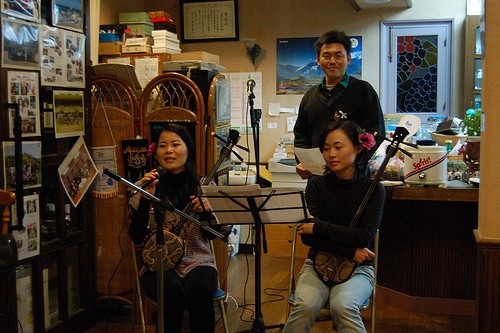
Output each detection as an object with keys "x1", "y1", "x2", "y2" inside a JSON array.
[{"x1": 98, "y1": 11, "x2": 181, "y2": 56}]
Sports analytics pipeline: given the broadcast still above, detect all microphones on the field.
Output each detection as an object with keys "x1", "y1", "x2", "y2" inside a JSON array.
[
  {"x1": 127, "y1": 166, "x2": 167, "y2": 196},
  {"x1": 247, "y1": 79, "x2": 255, "y2": 97}
]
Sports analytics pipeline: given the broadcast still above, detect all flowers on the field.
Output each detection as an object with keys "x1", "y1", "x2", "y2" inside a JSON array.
[
  {"x1": 147, "y1": 142, "x2": 156, "y2": 156},
  {"x1": 359, "y1": 132, "x2": 376, "y2": 150}
]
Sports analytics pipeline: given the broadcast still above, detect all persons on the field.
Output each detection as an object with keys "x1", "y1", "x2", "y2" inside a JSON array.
[
  {"x1": 283, "y1": 120, "x2": 385, "y2": 332},
  {"x1": 18, "y1": 96, "x2": 28, "y2": 112},
  {"x1": 128, "y1": 123, "x2": 218, "y2": 333},
  {"x1": 58, "y1": 108, "x2": 81, "y2": 126},
  {"x1": 10, "y1": 158, "x2": 38, "y2": 187},
  {"x1": 68, "y1": 160, "x2": 90, "y2": 198},
  {"x1": 293, "y1": 30, "x2": 386, "y2": 180}
]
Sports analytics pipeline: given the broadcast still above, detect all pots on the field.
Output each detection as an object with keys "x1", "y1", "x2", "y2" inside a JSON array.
[{"x1": 403, "y1": 146, "x2": 448, "y2": 189}]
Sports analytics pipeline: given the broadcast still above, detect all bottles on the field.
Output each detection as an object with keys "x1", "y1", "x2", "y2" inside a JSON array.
[{"x1": 447, "y1": 155, "x2": 470, "y2": 184}]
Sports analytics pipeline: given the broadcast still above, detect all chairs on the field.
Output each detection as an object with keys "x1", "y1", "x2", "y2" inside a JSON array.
[
  {"x1": 284, "y1": 225, "x2": 379, "y2": 333},
  {"x1": 131, "y1": 239, "x2": 228, "y2": 333}
]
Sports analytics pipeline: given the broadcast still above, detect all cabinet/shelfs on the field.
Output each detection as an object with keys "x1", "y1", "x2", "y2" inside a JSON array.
[{"x1": 0, "y1": 85, "x2": 97, "y2": 333}]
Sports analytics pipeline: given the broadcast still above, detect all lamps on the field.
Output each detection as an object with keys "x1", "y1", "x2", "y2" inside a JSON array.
[{"x1": 242, "y1": 38, "x2": 260, "y2": 66}]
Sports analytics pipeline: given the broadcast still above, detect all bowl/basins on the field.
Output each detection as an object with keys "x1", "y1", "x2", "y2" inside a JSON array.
[
  {"x1": 469, "y1": 178, "x2": 479, "y2": 188},
  {"x1": 428, "y1": 129, "x2": 466, "y2": 148}
]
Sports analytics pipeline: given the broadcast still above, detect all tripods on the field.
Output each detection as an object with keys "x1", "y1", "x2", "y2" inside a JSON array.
[{"x1": 200, "y1": 190, "x2": 308, "y2": 333}]
